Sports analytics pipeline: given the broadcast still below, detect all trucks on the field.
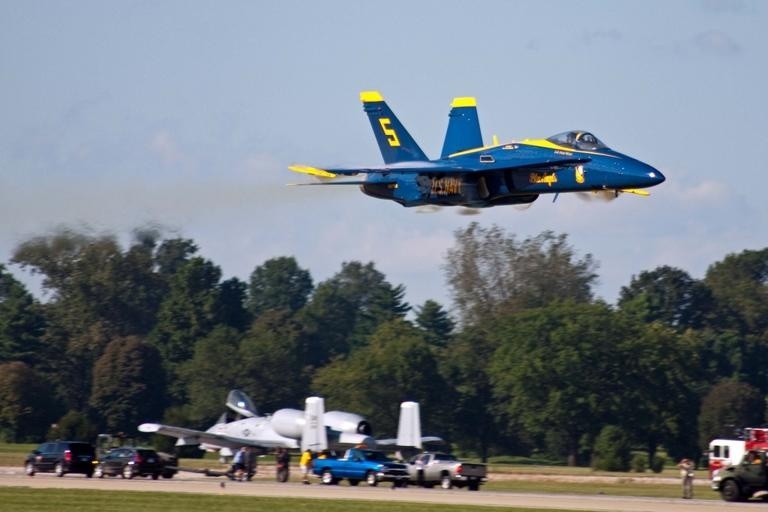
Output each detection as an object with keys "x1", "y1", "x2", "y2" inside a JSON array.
[{"x1": 711, "y1": 449, "x2": 768, "y2": 501}]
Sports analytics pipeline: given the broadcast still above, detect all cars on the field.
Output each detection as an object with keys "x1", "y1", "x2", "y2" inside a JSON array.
[{"x1": 24, "y1": 441, "x2": 178, "y2": 480}]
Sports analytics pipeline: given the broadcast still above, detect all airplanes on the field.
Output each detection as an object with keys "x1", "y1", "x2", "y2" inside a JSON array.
[
  {"x1": 287, "y1": 90, "x2": 665, "y2": 215},
  {"x1": 137, "y1": 389, "x2": 446, "y2": 482}
]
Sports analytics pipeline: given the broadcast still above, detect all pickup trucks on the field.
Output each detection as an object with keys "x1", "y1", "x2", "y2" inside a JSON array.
[{"x1": 312, "y1": 448, "x2": 488, "y2": 490}]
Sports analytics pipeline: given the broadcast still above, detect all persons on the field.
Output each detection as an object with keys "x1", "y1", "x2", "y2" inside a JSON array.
[
  {"x1": 678, "y1": 457, "x2": 694, "y2": 498},
  {"x1": 300, "y1": 449, "x2": 312, "y2": 484},
  {"x1": 317, "y1": 450, "x2": 326, "y2": 459},
  {"x1": 274, "y1": 445, "x2": 290, "y2": 482},
  {"x1": 228, "y1": 446, "x2": 256, "y2": 481}
]
